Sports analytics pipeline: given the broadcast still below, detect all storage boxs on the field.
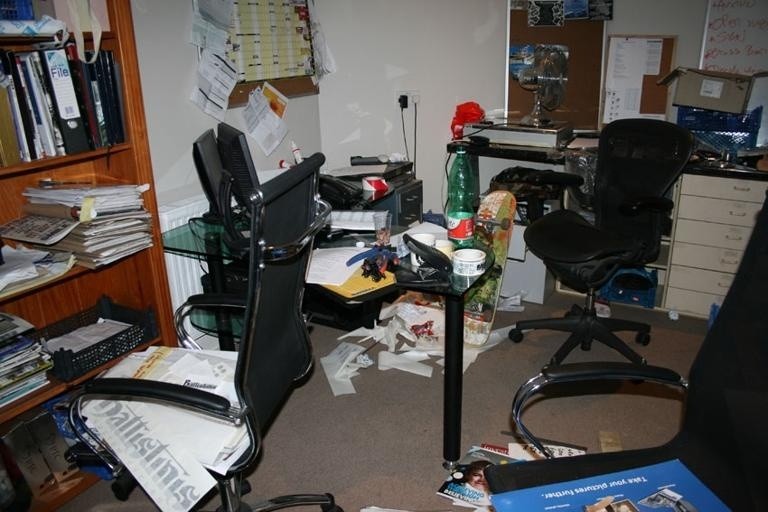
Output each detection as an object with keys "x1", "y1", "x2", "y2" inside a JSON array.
[{"x1": 656, "y1": 66, "x2": 768, "y2": 114}]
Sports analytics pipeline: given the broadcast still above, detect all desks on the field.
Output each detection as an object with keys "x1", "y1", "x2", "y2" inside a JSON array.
[
  {"x1": 161, "y1": 220, "x2": 496, "y2": 471},
  {"x1": 345, "y1": 178, "x2": 423, "y2": 226}
]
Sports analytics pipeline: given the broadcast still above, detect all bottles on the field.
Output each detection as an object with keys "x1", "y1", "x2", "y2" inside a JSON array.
[
  {"x1": 447, "y1": 191, "x2": 476, "y2": 248},
  {"x1": 447, "y1": 147, "x2": 477, "y2": 211}
]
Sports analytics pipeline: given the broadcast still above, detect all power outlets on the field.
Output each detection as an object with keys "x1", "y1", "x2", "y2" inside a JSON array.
[{"x1": 395, "y1": 90, "x2": 418, "y2": 105}]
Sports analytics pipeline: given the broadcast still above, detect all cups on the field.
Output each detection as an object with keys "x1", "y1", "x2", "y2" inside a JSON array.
[
  {"x1": 372, "y1": 211, "x2": 393, "y2": 245},
  {"x1": 435, "y1": 239, "x2": 454, "y2": 261},
  {"x1": 408, "y1": 233, "x2": 436, "y2": 267}
]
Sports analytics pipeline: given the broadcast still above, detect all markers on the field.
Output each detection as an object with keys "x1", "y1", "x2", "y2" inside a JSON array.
[
  {"x1": 290, "y1": 140, "x2": 303, "y2": 164},
  {"x1": 279, "y1": 160, "x2": 295, "y2": 169}
]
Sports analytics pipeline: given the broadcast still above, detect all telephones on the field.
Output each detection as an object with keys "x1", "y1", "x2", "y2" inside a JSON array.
[{"x1": 317, "y1": 174, "x2": 363, "y2": 209}]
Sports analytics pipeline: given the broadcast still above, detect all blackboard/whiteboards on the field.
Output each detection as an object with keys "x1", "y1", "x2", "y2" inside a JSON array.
[{"x1": 698, "y1": 0, "x2": 767, "y2": 149}]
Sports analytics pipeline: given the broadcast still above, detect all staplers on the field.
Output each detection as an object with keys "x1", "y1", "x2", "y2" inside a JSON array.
[{"x1": 395, "y1": 233, "x2": 453, "y2": 289}]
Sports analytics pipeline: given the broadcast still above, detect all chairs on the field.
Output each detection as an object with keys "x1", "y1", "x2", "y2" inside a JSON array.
[
  {"x1": 484, "y1": 185, "x2": 768, "y2": 512},
  {"x1": 508, "y1": 118, "x2": 693, "y2": 372},
  {"x1": 64, "y1": 152, "x2": 344, "y2": 512}
]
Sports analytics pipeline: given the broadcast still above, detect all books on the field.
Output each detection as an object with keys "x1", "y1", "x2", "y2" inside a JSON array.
[
  {"x1": 0, "y1": 313, "x2": 54, "y2": 408},
  {"x1": 1, "y1": 181, "x2": 154, "y2": 296},
  {"x1": 0, "y1": 45, "x2": 125, "y2": 167},
  {"x1": 437, "y1": 443, "x2": 586, "y2": 508}
]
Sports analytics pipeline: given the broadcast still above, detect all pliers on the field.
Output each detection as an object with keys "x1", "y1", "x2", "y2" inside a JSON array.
[{"x1": 346, "y1": 244, "x2": 398, "y2": 273}]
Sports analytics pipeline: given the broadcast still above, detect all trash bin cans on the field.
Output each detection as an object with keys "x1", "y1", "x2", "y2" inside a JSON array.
[{"x1": 488, "y1": 167, "x2": 585, "y2": 305}]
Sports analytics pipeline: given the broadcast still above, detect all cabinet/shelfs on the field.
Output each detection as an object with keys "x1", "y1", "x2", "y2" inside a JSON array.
[
  {"x1": 446, "y1": 139, "x2": 768, "y2": 321},
  {"x1": 0, "y1": 0, "x2": 178, "y2": 512}
]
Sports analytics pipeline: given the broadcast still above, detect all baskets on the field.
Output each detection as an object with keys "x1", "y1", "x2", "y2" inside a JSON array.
[
  {"x1": 25, "y1": 292, "x2": 161, "y2": 384},
  {"x1": 677, "y1": 105, "x2": 763, "y2": 153}
]
